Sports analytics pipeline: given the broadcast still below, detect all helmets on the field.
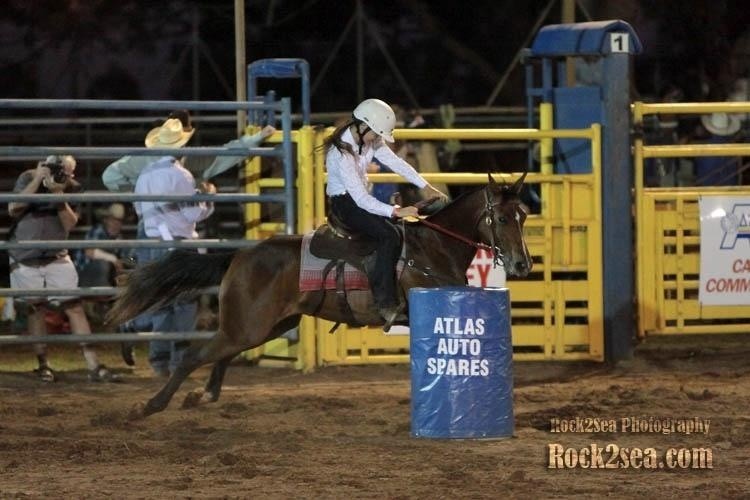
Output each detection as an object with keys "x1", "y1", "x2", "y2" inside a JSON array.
[{"x1": 353, "y1": 98, "x2": 396, "y2": 143}]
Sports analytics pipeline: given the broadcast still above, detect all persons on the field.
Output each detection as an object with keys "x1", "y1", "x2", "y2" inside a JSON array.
[
  {"x1": 8, "y1": 110, "x2": 275, "y2": 382},
  {"x1": 659, "y1": 78, "x2": 750, "y2": 186},
  {"x1": 326, "y1": 99, "x2": 449, "y2": 323}
]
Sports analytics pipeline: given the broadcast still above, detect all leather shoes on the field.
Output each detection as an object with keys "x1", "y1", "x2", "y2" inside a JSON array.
[
  {"x1": 378, "y1": 306, "x2": 408, "y2": 322},
  {"x1": 115, "y1": 323, "x2": 136, "y2": 365}
]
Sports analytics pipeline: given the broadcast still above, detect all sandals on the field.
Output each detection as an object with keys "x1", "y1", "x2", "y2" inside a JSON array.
[
  {"x1": 88, "y1": 364, "x2": 125, "y2": 383},
  {"x1": 39, "y1": 365, "x2": 55, "y2": 382}
]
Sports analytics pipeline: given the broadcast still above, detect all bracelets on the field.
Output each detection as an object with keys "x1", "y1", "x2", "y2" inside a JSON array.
[{"x1": 391, "y1": 206, "x2": 400, "y2": 217}]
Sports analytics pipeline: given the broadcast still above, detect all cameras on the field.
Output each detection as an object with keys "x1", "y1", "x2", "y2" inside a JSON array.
[{"x1": 41, "y1": 158, "x2": 67, "y2": 184}]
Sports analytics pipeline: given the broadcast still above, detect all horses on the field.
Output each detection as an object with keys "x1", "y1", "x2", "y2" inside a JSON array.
[{"x1": 97, "y1": 168, "x2": 535, "y2": 421}]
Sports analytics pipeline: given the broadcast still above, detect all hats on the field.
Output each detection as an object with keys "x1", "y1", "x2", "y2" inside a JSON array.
[
  {"x1": 96, "y1": 204, "x2": 125, "y2": 221},
  {"x1": 700, "y1": 113, "x2": 741, "y2": 136},
  {"x1": 145, "y1": 118, "x2": 195, "y2": 150}
]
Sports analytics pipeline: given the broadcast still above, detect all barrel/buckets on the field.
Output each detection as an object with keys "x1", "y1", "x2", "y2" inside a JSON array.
[{"x1": 409, "y1": 285, "x2": 513, "y2": 440}]
